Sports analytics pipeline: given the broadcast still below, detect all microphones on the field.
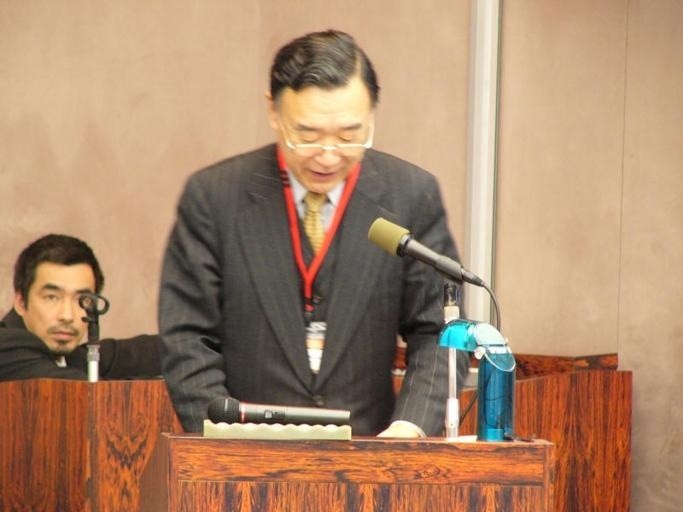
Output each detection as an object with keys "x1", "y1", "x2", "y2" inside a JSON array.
[
  {"x1": 208, "y1": 397, "x2": 352, "y2": 424},
  {"x1": 367, "y1": 217, "x2": 484, "y2": 288}
]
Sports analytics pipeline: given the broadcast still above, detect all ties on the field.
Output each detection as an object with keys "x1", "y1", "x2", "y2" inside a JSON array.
[{"x1": 304, "y1": 193, "x2": 328, "y2": 253}]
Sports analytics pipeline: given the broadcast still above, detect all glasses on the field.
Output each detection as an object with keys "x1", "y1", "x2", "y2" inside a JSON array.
[{"x1": 277, "y1": 114, "x2": 374, "y2": 156}]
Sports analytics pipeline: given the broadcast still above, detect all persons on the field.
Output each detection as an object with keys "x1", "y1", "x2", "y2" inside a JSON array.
[
  {"x1": 2, "y1": 232, "x2": 163, "y2": 381},
  {"x1": 157, "y1": 30, "x2": 472, "y2": 442}
]
